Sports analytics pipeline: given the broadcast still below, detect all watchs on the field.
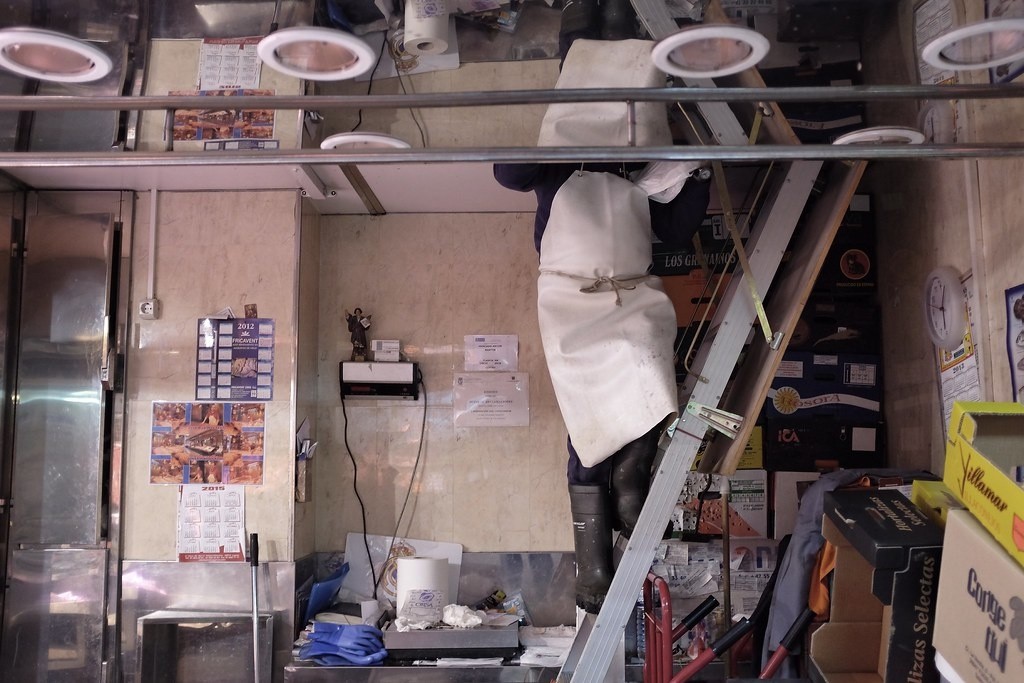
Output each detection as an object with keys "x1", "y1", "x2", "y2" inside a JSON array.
[{"x1": 693, "y1": 166, "x2": 713, "y2": 181}]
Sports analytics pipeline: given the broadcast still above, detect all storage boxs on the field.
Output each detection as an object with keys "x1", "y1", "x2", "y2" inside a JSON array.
[
  {"x1": 809, "y1": 489, "x2": 945, "y2": 683},
  {"x1": 764, "y1": 418, "x2": 887, "y2": 472},
  {"x1": 766, "y1": 352, "x2": 882, "y2": 418},
  {"x1": 932, "y1": 509, "x2": 1024, "y2": 683},
  {"x1": 910, "y1": 480, "x2": 963, "y2": 528},
  {"x1": 767, "y1": 472, "x2": 820, "y2": 540},
  {"x1": 942, "y1": 401, "x2": 1024, "y2": 568},
  {"x1": 765, "y1": 77, "x2": 867, "y2": 144},
  {"x1": 753, "y1": 12, "x2": 861, "y2": 70},
  {"x1": 663, "y1": 269, "x2": 759, "y2": 328},
  {"x1": 692, "y1": 426, "x2": 763, "y2": 471},
  {"x1": 786, "y1": 291, "x2": 882, "y2": 355},
  {"x1": 771, "y1": 241, "x2": 878, "y2": 293},
  {"x1": 649, "y1": 213, "x2": 754, "y2": 276},
  {"x1": 759, "y1": 191, "x2": 877, "y2": 244},
  {"x1": 672, "y1": 471, "x2": 768, "y2": 539}
]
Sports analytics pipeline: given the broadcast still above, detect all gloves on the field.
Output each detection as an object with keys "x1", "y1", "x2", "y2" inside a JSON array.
[
  {"x1": 299, "y1": 643, "x2": 388, "y2": 665},
  {"x1": 306, "y1": 622, "x2": 383, "y2": 653}
]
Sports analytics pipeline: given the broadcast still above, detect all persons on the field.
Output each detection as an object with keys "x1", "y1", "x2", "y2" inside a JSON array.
[
  {"x1": 492, "y1": 160, "x2": 716, "y2": 615},
  {"x1": 344, "y1": 307, "x2": 372, "y2": 362}
]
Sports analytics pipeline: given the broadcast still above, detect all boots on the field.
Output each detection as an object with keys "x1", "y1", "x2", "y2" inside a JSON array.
[
  {"x1": 611, "y1": 412, "x2": 674, "y2": 540},
  {"x1": 568, "y1": 485, "x2": 615, "y2": 615}
]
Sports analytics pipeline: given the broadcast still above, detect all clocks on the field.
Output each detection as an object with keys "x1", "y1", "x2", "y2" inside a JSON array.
[{"x1": 922, "y1": 266, "x2": 968, "y2": 350}]
